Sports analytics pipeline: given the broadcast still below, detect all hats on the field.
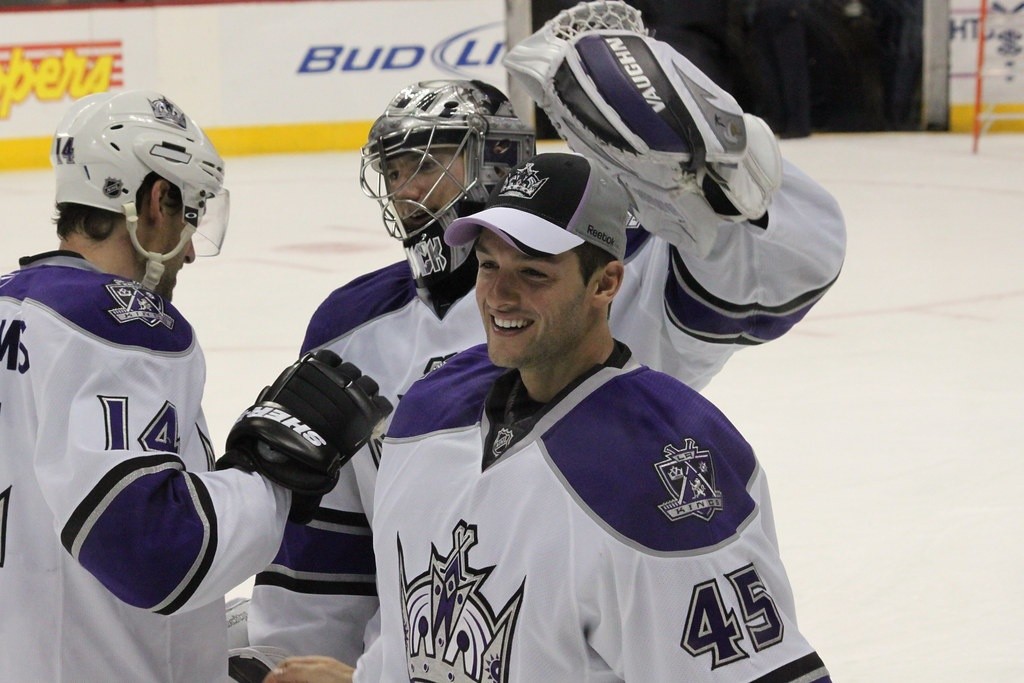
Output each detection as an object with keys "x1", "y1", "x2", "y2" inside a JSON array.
[{"x1": 442, "y1": 154, "x2": 630, "y2": 263}]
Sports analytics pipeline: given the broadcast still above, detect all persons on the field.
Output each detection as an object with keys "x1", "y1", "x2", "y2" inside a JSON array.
[
  {"x1": 247, "y1": 0, "x2": 846, "y2": 683},
  {"x1": 0, "y1": 86, "x2": 395, "y2": 683},
  {"x1": 263, "y1": 152, "x2": 831, "y2": 683}
]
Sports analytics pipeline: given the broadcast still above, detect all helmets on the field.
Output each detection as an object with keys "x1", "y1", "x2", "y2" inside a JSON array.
[
  {"x1": 361, "y1": 78, "x2": 536, "y2": 279},
  {"x1": 51, "y1": 90, "x2": 224, "y2": 228}
]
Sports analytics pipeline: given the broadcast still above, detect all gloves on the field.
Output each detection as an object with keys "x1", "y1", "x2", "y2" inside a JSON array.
[{"x1": 226, "y1": 351, "x2": 393, "y2": 523}]
